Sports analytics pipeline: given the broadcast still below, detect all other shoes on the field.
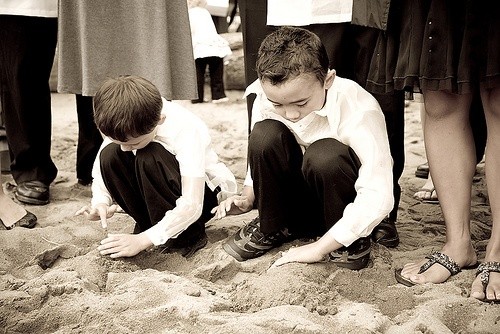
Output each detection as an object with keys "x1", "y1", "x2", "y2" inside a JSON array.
[
  {"x1": 167, "y1": 238, "x2": 207, "y2": 259},
  {"x1": 415, "y1": 162, "x2": 431, "y2": 178},
  {"x1": 373, "y1": 222, "x2": 400, "y2": 247}
]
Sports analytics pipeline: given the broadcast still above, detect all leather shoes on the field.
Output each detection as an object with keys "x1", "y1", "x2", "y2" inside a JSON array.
[{"x1": 15, "y1": 180, "x2": 50, "y2": 204}]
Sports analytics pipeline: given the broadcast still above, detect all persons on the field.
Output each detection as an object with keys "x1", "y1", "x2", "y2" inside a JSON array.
[
  {"x1": 187, "y1": 0, "x2": 238, "y2": 105},
  {"x1": 0, "y1": 154, "x2": 38, "y2": 231},
  {"x1": 54, "y1": 0, "x2": 199, "y2": 186},
  {"x1": 211, "y1": 25, "x2": 397, "y2": 271},
  {"x1": 70, "y1": 74, "x2": 239, "y2": 260},
  {"x1": 350, "y1": 0, "x2": 500, "y2": 305},
  {"x1": 237, "y1": 0, "x2": 407, "y2": 248},
  {"x1": 0, "y1": 0, "x2": 61, "y2": 205}
]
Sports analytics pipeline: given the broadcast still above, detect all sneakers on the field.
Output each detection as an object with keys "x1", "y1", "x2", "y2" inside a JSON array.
[
  {"x1": 328, "y1": 236, "x2": 371, "y2": 269},
  {"x1": 223, "y1": 217, "x2": 297, "y2": 260}
]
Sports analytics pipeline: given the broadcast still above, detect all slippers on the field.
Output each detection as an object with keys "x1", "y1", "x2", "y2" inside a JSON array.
[
  {"x1": 474, "y1": 262, "x2": 500, "y2": 304},
  {"x1": 395, "y1": 251, "x2": 479, "y2": 288},
  {"x1": 416, "y1": 187, "x2": 440, "y2": 203}
]
